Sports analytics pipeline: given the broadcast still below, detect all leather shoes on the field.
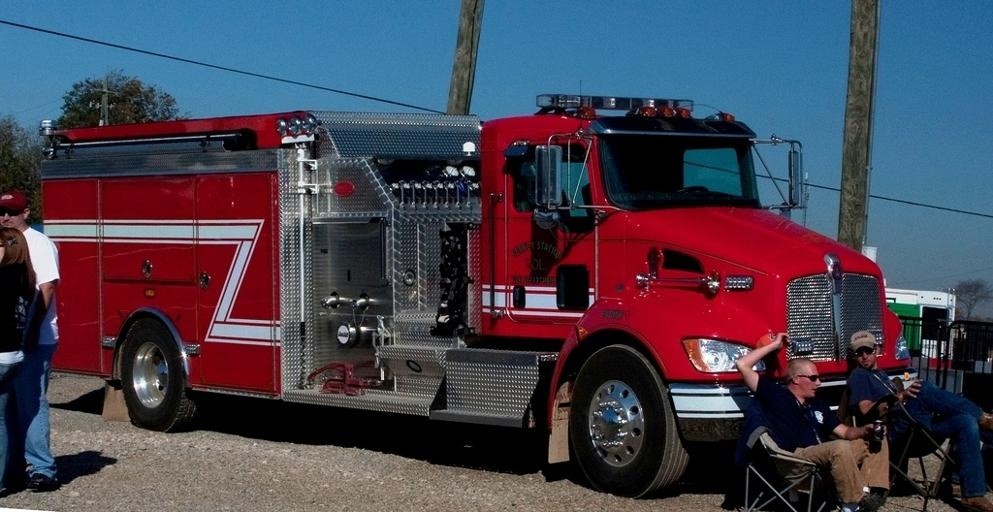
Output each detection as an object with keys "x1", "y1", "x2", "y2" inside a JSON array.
[
  {"x1": 940, "y1": 480, "x2": 993, "y2": 512},
  {"x1": 24, "y1": 473, "x2": 61, "y2": 490},
  {"x1": 978, "y1": 411, "x2": 993, "y2": 432}
]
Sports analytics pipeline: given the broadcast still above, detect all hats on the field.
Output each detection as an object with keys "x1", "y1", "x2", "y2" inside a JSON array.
[
  {"x1": 848, "y1": 331, "x2": 876, "y2": 353},
  {"x1": 0, "y1": 190, "x2": 28, "y2": 214}
]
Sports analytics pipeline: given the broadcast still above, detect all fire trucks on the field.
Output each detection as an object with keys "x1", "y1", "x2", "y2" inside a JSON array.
[{"x1": 40, "y1": 94, "x2": 918, "y2": 498}]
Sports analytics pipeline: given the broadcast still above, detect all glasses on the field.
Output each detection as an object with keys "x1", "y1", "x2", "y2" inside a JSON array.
[
  {"x1": 854, "y1": 348, "x2": 876, "y2": 357},
  {"x1": 786, "y1": 374, "x2": 823, "y2": 382}
]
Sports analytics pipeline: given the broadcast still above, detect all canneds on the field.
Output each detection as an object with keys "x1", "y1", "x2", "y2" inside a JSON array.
[{"x1": 874, "y1": 420, "x2": 884, "y2": 442}]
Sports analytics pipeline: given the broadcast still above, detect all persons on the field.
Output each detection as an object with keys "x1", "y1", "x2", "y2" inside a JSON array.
[
  {"x1": 735, "y1": 331, "x2": 892, "y2": 512},
  {"x1": 0, "y1": 226, "x2": 42, "y2": 497},
  {"x1": 844, "y1": 331, "x2": 993, "y2": 512},
  {"x1": 0, "y1": 189, "x2": 63, "y2": 491}
]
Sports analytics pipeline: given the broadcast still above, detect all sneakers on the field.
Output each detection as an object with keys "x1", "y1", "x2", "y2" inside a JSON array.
[{"x1": 838, "y1": 490, "x2": 882, "y2": 512}]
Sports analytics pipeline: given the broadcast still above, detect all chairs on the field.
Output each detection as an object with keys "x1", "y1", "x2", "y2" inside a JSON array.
[{"x1": 747, "y1": 376, "x2": 936, "y2": 512}]
